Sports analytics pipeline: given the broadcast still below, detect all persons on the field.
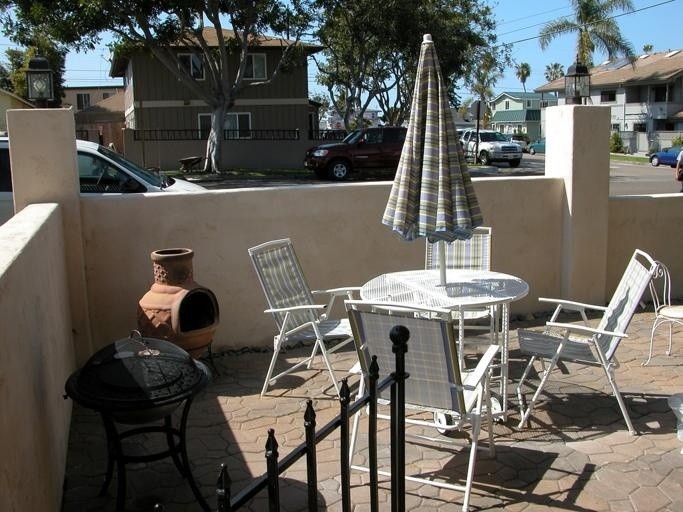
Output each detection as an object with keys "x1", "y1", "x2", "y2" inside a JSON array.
[{"x1": 675, "y1": 150, "x2": 683, "y2": 193}]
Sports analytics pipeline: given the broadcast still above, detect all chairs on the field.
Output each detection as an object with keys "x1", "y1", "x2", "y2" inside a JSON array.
[
  {"x1": 247, "y1": 237, "x2": 363, "y2": 401},
  {"x1": 514, "y1": 246, "x2": 658, "y2": 435},
  {"x1": 644, "y1": 259, "x2": 682, "y2": 365},
  {"x1": 342, "y1": 300, "x2": 501, "y2": 512},
  {"x1": 421, "y1": 226, "x2": 498, "y2": 376}
]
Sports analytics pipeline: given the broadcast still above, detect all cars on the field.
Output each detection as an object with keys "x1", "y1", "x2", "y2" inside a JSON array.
[
  {"x1": 455, "y1": 128, "x2": 469, "y2": 140},
  {"x1": 527, "y1": 139, "x2": 545, "y2": 156},
  {"x1": 649, "y1": 145, "x2": 683, "y2": 168},
  {"x1": 0, "y1": 133, "x2": 210, "y2": 226}
]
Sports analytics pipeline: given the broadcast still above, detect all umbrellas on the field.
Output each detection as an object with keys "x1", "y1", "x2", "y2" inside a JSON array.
[{"x1": 379, "y1": 33, "x2": 484, "y2": 285}]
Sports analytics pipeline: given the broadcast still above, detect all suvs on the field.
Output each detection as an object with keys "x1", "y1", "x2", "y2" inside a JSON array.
[
  {"x1": 461, "y1": 128, "x2": 524, "y2": 169},
  {"x1": 304, "y1": 127, "x2": 409, "y2": 181}
]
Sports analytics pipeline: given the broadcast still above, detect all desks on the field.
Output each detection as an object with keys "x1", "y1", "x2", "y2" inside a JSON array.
[{"x1": 358, "y1": 267, "x2": 530, "y2": 433}]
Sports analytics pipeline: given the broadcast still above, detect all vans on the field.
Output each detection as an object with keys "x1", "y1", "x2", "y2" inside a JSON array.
[{"x1": 509, "y1": 135, "x2": 528, "y2": 151}]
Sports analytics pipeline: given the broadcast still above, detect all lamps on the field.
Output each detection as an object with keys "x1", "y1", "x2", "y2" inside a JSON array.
[
  {"x1": 25, "y1": 33, "x2": 59, "y2": 108},
  {"x1": 564, "y1": 53, "x2": 590, "y2": 103}
]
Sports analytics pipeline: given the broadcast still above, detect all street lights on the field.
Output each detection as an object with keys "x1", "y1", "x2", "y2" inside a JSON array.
[
  {"x1": 25, "y1": 52, "x2": 57, "y2": 110},
  {"x1": 564, "y1": 55, "x2": 592, "y2": 107}
]
